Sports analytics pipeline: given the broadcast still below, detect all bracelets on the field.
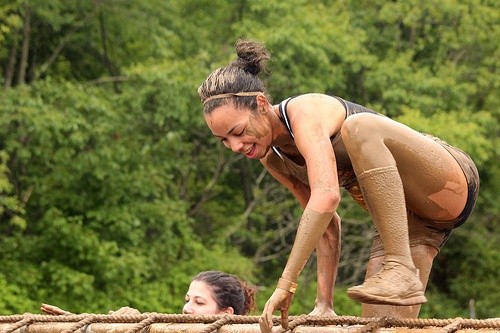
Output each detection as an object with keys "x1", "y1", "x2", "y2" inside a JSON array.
[{"x1": 275, "y1": 278, "x2": 298, "y2": 294}]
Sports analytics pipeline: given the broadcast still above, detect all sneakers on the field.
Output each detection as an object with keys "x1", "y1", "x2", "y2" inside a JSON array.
[{"x1": 346, "y1": 259, "x2": 427, "y2": 305}]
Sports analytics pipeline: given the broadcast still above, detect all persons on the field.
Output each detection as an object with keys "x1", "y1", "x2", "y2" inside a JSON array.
[
  {"x1": 195, "y1": 37, "x2": 481, "y2": 333},
  {"x1": 41, "y1": 270, "x2": 257, "y2": 316}
]
